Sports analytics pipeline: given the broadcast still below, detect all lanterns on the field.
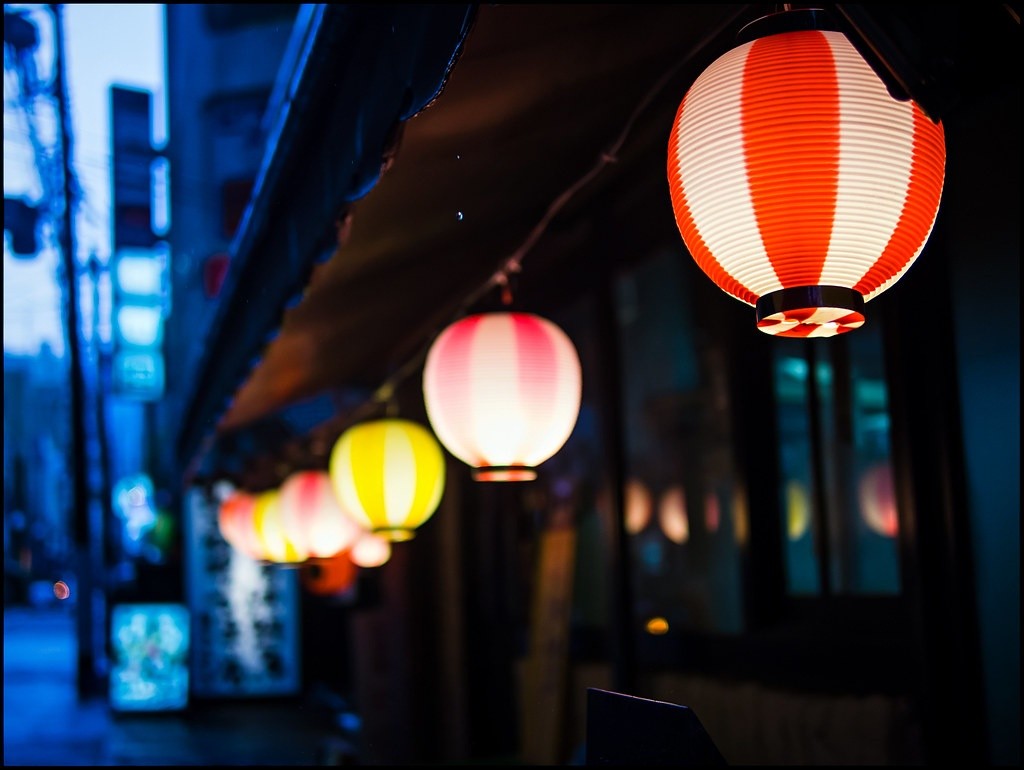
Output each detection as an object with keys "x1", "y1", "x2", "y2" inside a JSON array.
[
  {"x1": 216, "y1": 420, "x2": 447, "y2": 569},
  {"x1": 667, "y1": 0, "x2": 948, "y2": 338},
  {"x1": 422, "y1": 313, "x2": 584, "y2": 482}
]
